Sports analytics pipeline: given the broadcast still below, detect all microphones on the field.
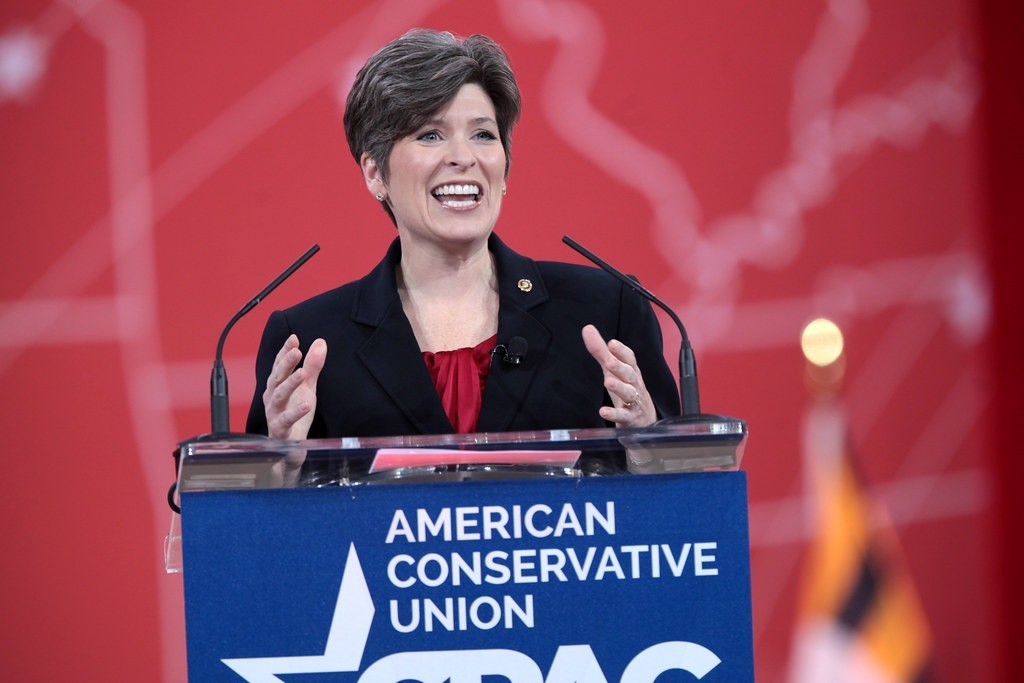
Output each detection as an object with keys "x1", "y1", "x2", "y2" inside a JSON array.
[
  {"x1": 178, "y1": 244, "x2": 320, "y2": 448},
  {"x1": 508, "y1": 336, "x2": 528, "y2": 366},
  {"x1": 562, "y1": 235, "x2": 730, "y2": 426}
]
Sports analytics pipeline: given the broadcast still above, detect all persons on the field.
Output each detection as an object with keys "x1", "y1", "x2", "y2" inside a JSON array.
[{"x1": 245, "y1": 28, "x2": 683, "y2": 488}]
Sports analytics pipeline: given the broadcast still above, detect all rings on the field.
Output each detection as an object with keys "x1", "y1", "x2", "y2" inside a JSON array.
[{"x1": 623, "y1": 389, "x2": 642, "y2": 407}]
